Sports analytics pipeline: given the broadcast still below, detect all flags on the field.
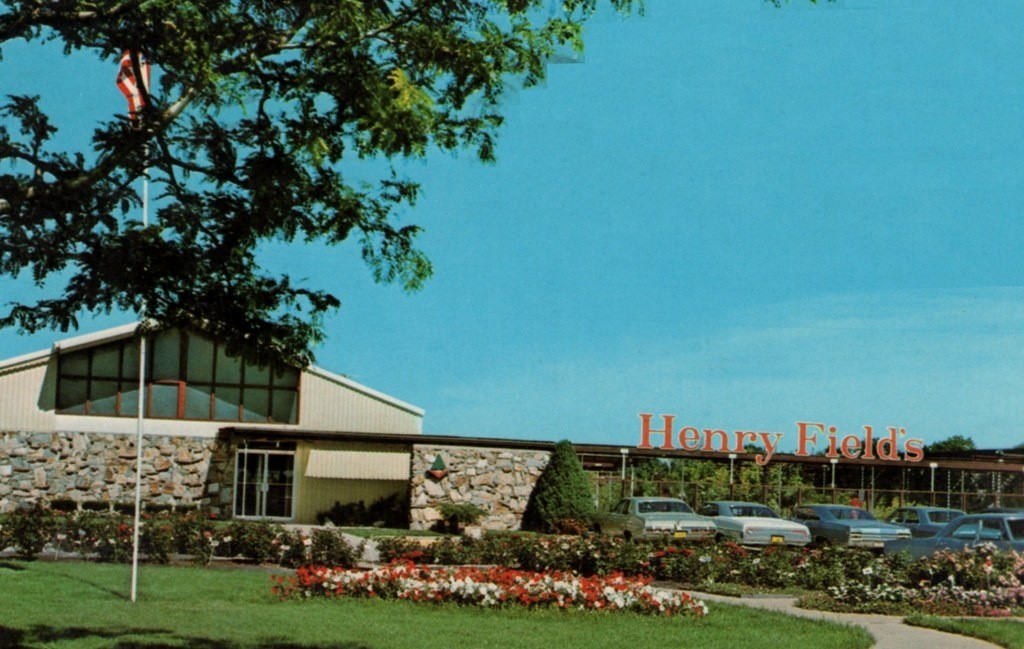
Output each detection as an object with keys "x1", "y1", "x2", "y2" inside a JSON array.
[{"x1": 116, "y1": 48, "x2": 150, "y2": 120}]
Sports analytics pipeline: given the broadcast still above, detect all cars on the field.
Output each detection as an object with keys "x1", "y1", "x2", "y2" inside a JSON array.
[
  {"x1": 883, "y1": 513, "x2": 1023, "y2": 555},
  {"x1": 698, "y1": 500, "x2": 811, "y2": 550},
  {"x1": 603, "y1": 496, "x2": 718, "y2": 544},
  {"x1": 882, "y1": 506, "x2": 1002, "y2": 542},
  {"x1": 784, "y1": 502, "x2": 911, "y2": 554}
]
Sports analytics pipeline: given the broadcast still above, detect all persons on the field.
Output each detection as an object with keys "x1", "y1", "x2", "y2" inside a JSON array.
[{"x1": 849, "y1": 493, "x2": 863, "y2": 520}]
